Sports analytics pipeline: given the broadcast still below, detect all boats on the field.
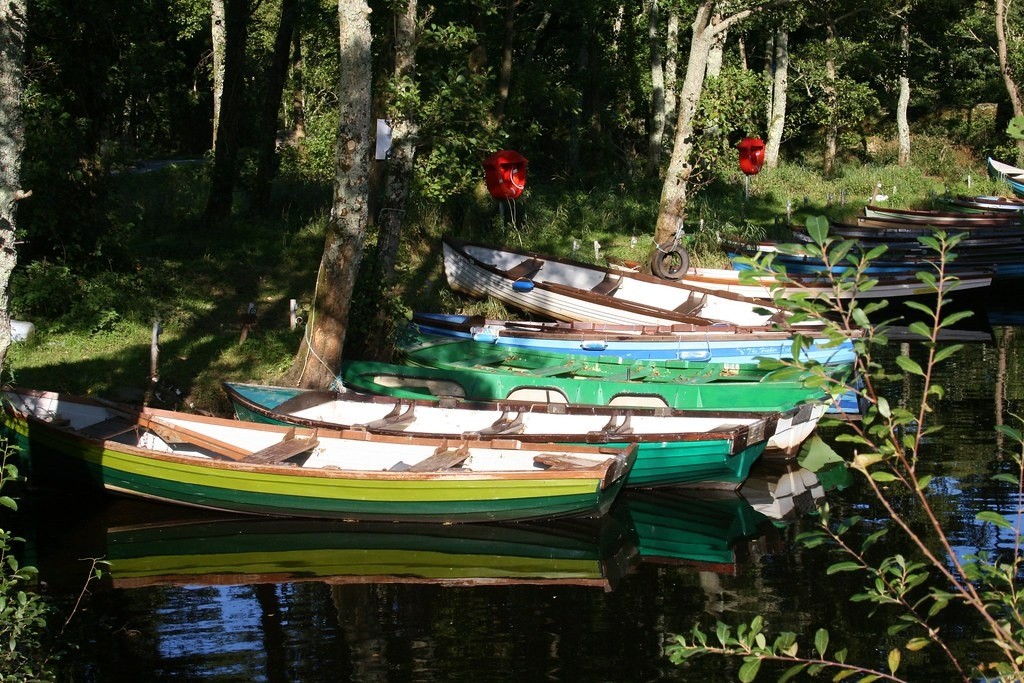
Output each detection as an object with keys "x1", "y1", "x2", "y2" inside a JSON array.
[
  {"x1": 744, "y1": 416, "x2": 854, "y2": 528},
  {"x1": 847, "y1": 301, "x2": 994, "y2": 345},
  {"x1": 101, "y1": 516, "x2": 639, "y2": 593},
  {"x1": 225, "y1": 377, "x2": 780, "y2": 493},
  {"x1": 622, "y1": 492, "x2": 786, "y2": 578},
  {"x1": 2, "y1": 384, "x2": 639, "y2": 524}
]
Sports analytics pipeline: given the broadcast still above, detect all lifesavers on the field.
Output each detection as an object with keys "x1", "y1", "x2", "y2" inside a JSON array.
[{"x1": 651, "y1": 242, "x2": 690, "y2": 282}]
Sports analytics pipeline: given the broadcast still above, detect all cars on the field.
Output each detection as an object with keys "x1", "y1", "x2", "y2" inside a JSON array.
[{"x1": 343, "y1": 157, "x2": 1024, "y2": 501}]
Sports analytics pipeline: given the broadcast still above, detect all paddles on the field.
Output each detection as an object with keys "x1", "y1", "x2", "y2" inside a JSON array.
[
  {"x1": 541, "y1": 281, "x2": 731, "y2": 323},
  {"x1": 89, "y1": 393, "x2": 253, "y2": 459},
  {"x1": 505, "y1": 322, "x2": 630, "y2": 335}
]
